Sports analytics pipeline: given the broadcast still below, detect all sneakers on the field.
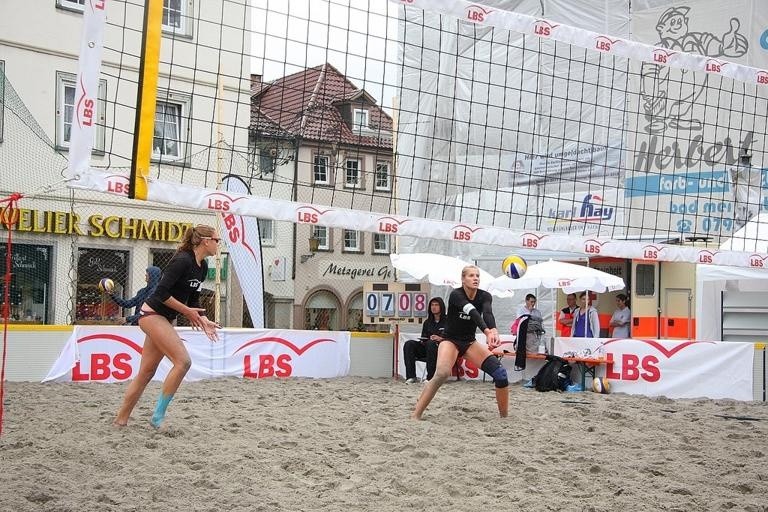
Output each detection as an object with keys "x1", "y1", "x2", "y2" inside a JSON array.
[{"x1": 405, "y1": 378, "x2": 428, "y2": 385}]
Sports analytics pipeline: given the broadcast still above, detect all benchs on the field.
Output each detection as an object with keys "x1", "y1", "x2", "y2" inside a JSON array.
[{"x1": 483, "y1": 352, "x2": 613, "y2": 392}]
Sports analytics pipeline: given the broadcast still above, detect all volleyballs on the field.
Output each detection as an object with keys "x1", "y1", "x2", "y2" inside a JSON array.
[
  {"x1": 502, "y1": 255, "x2": 527, "y2": 279},
  {"x1": 592, "y1": 377, "x2": 610, "y2": 393},
  {"x1": 99, "y1": 278, "x2": 114, "y2": 292}
]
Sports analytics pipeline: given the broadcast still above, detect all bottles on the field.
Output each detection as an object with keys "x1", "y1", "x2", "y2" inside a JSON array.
[
  {"x1": 538, "y1": 332, "x2": 546, "y2": 354},
  {"x1": 598, "y1": 342, "x2": 604, "y2": 359}
]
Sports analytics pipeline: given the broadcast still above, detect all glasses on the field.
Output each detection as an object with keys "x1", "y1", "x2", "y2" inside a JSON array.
[{"x1": 199, "y1": 235, "x2": 220, "y2": 244}]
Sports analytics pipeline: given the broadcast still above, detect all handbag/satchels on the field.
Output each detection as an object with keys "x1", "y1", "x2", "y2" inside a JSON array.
[{"x1": 513, "y1": 314, "x2": 545, "y2": 354}]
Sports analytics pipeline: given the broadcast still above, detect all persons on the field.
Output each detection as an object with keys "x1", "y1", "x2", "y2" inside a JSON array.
[
  {"x1": 516, "y1": 293, "x2": 543, "y2": 319},
  {"x1": 115, "y1": 224, "x2": 222, "y2": 428},
  {"x1": 557, "y1": 292, "x2": 580, "y2": 336},
  {"x1": 569, "y1": 290, "x2": 600, "y2": 338},
  {"x1": 410, "y1": 265, "x2": 510, "y2": 418},
  {"x1": 609, "y1": 293, "x2": 630, "y2": 337},
  {"x1": 107, "y1": 264, "x2": 161, "y2": 325},
  {"x1": 403, "y1": 296, "x2": 448, "y2": 385}
]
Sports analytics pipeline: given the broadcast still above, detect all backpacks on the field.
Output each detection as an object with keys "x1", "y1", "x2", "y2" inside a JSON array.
[{"x1": 536, "y1": 356, "x2": 572, "y2": 392}]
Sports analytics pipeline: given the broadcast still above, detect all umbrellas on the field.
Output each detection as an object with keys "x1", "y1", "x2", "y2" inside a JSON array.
[
  {"x1": 390, "y1": 251, "x2": 514, "y2": 307},
  {"x1": 488, "y1": 257, "x2": 626, "y2": 337}
]
